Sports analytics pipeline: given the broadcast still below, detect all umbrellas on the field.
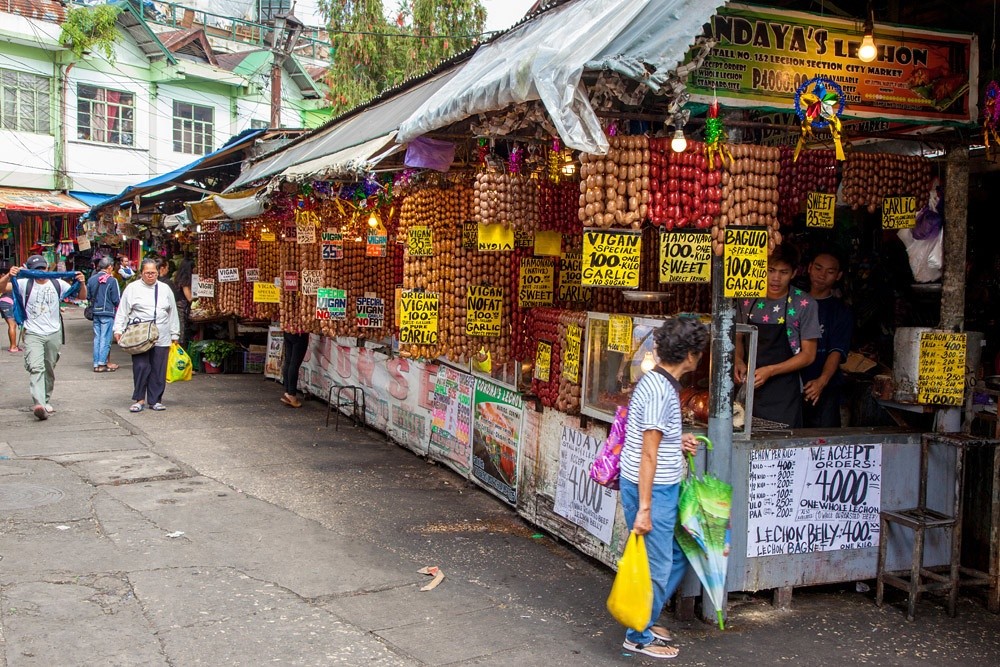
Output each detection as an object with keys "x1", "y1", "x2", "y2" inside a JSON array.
[{"x1": 673, "y1": 436, "x2": 735, "y2": 631}]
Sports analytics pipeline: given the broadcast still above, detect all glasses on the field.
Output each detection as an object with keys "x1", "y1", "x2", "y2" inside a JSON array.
[{"x1": 142, "y1": 271, "x2": 157, "y2": 276}]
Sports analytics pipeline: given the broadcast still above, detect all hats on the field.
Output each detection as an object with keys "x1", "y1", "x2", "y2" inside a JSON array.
[{"x1": 26, "y1": 255, "x2": 47, "y2": 270}]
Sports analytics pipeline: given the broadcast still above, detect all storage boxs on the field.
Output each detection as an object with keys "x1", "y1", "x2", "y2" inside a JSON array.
[{"x1": 228, "y1": 350, "x2": 267, "y2": 374}]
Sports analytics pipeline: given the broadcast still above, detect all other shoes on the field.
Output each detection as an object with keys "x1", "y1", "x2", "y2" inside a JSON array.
[
  {"x1": 46, "y1": 404, "x2": 53, "y2": 412},
  {"x1": 34, "y1": 406, "x2": 47, "y2": 420}
]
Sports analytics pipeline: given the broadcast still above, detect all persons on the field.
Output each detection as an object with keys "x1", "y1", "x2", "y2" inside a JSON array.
[
  {"x1": 280, "y1": 330, "x2": 310, "y2": 407},
  {"x1": 618, "y1": 315, "x2": 710, "y2": 658},
  {"x1": 90, "y1": 250, "x2": 199, "y2": 351},
  {"x1": 732, "y1": 247, "x2": 822, "y2": 431},
  {"x1": 87, "y1": 257, "x2": 121, "y2": 373},
  {"x1": 0, "y1": 254, "x2": 87, "y2": 420},
  {"x1": 801, "y1": 248, "x2": 854, "y2": 427},
  {"x1": 112, "y1": 257, "x2": 181, "y2": 412}
]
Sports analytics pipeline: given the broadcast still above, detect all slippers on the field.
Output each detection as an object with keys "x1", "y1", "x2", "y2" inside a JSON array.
[
  {"x1": 150, "y1": 402, "x2": 165, "y2": 410},
  {"x1": 131, "y1": 403, "x2": 144, "y2": 412},
  {"x1": 650, "y1": 622, "x2": 672, "y2": 642},
  {"x1": 623, "y1": 639, "x2": 677, "y2": 658},
  {"x1": 281, "y1": 398, "x2": 301, "y2": 407}
]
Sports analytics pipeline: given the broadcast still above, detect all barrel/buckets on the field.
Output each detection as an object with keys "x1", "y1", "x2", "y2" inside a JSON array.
[{"x1": 204, "y1": 361, "x2": 219, "y2": 373}]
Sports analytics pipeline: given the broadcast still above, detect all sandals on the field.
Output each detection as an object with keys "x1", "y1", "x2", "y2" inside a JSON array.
[{"x1": 94, "y1": 365, "x2": 115, "y2": 372}]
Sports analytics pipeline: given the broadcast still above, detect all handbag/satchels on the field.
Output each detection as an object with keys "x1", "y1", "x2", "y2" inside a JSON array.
[
  {"x1": 165, "y1": 343, "x2": 193, "y2": 383},
  {"x1": 590, "y1": 406, "x2": 630, "y2": 490},
  {"x1": 117, "y1": 321, "x2": 159, "y2": 355},
  {"x1": 606, "y1": 531, "x2": 653, "y2": 632},
  {"x1": 84, "y1": 305, "x2": 93, "y2": 320}
]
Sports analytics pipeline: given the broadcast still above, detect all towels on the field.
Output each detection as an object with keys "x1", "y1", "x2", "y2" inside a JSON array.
[{"x1": 11, "y1": 269, "x2": 81, "y2": 326}]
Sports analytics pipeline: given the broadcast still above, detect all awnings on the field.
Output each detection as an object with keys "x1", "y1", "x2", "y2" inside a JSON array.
[{"x1": 1, "y1": 186, "x2": 115, "y2": 214}]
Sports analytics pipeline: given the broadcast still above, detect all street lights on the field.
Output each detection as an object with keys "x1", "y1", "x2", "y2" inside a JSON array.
[{"x1": 267, "y1": 0, "x2": 305, "y2": 127}]
